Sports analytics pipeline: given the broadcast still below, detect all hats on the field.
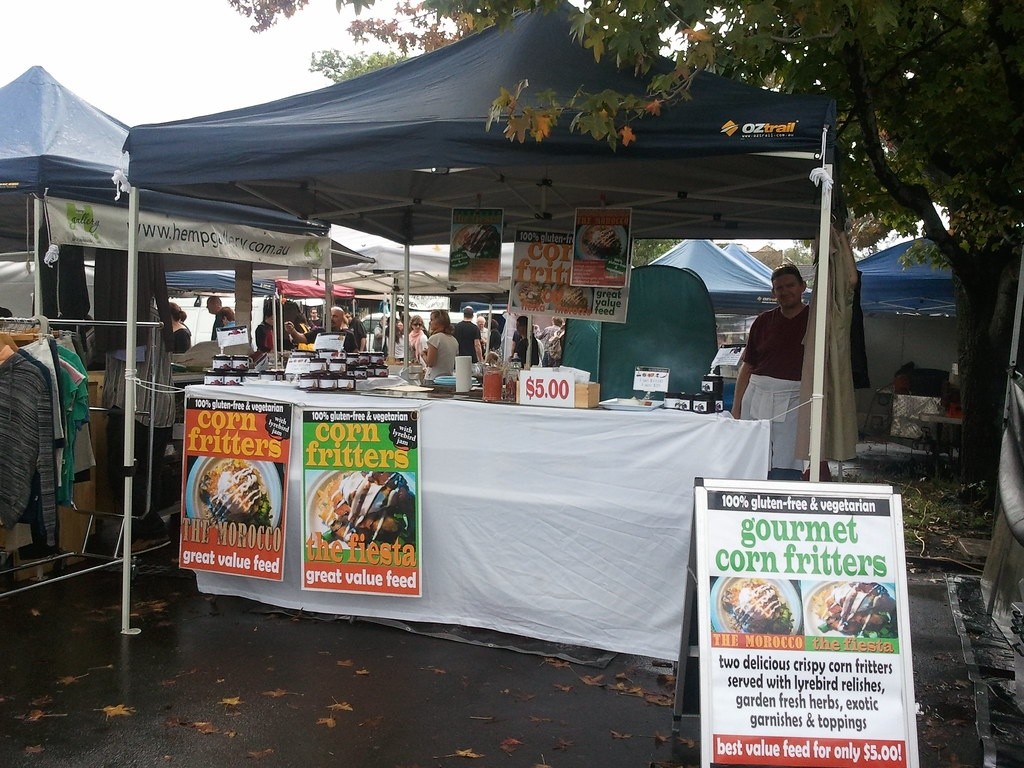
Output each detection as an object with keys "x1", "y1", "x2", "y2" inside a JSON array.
[{"x1": 463, "y1": 306, "x2": 474, "y2": 313}]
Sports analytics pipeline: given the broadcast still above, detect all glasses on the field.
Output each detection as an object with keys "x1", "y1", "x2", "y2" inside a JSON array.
[
  {"x1": 412, "y1": 323, "x2": 422, "y2": 327},
  {"x1": 346, "y1": 315, "x2": 351, "y2": 318}
]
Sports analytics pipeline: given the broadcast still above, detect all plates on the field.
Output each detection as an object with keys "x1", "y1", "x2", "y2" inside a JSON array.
[
  {"x1": 710, "y1": 577, "x2": 802, "y2": 635},
  {"x1": 186, "y1": 453, "x2": 283, "y2": 532},
  {"x1": 576, "y1": 223, "x2": 627, "y2": 261},
  {"x1": 803, "y1": 581, "x2": 898, "y2": 639},
  {"x1": 307, "y1": 469, "x2": 416, "y2": 552}
]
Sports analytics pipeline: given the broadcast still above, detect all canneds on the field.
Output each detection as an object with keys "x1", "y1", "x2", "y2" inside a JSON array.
[
  {"x1": 203, "y1": 352, "x2": 389, "y2": 390},
  {"x1": 664, "y1": 372, "x2": 725, "y2": 414}
]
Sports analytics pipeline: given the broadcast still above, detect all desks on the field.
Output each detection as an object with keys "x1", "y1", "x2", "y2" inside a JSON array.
[{"x1": 919, "y1": 413, "x2": 963, "y2": 478}]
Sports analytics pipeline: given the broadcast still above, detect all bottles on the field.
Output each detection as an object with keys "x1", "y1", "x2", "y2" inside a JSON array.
[{"x1": 505, "y1": 353, "x2": 520, "y2": 402}]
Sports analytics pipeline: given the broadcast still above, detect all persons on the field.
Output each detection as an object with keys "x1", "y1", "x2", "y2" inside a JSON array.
[
  {"x1": 168, "y1": 293, "x2": 368, "y2": 353},
  {"x1": 731, "y1": 264, "x2": 810, "y2": 481},
  {"x1": 373, "y1": 306, "x2": 568, "y2": 384}
]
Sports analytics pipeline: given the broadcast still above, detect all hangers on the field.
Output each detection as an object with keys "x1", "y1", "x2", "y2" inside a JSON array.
[{"x1": 0, "y1": 315, "x2": 77, "y2": 353}]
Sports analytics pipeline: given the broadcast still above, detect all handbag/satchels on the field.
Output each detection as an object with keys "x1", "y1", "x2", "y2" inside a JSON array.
[{"x1": 999, "y1": 368, "x2": 1024, "y2": 547}]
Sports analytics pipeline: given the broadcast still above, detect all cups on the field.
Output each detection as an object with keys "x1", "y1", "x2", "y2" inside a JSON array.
[
  {"x1": 453, "y1": 355, "x2": 473, "y2": 393},
  {"x1": 482, "y1": 366, "x2": 502, "y2": 402}
]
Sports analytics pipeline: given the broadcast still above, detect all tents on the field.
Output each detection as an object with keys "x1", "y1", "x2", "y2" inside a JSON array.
[
  {"x1": 0, "y1": 65, "x2": 332, "y2": 326},
  {"x1": 121, "y1": 0, "x2": 843, "y2": 643},
  {"x1": 838, "y1": 231, "x2": 955, "y2": 480},
  {"x1": 315, "y1": 224, "x2": 533, "y2": 367},
  {"x1": 163, "y1": 270, "x2": 355, "y2": 370},
  {"x1": 644, "y1": 239, "x2": 814, "y2": 315}
]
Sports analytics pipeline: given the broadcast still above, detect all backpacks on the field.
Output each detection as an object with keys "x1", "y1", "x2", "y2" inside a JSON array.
[{"x1": 548, "y1": 328, "x2": 566, "y2": 360}]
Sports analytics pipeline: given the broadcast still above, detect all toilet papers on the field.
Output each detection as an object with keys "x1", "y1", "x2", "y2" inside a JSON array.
[{"x1": 455, "y1": 356, "x2": 472, "y2": 392}]
